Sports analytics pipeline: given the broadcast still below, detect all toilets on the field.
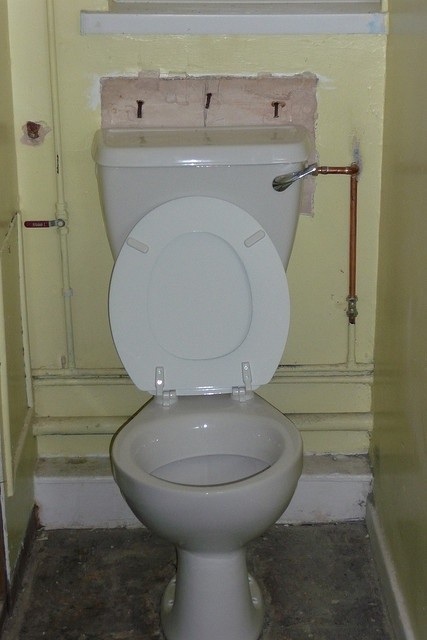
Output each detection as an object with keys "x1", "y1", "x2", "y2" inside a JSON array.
[{"x1": 91, "y1": 123, "x2": 309, "y2": 639}]
